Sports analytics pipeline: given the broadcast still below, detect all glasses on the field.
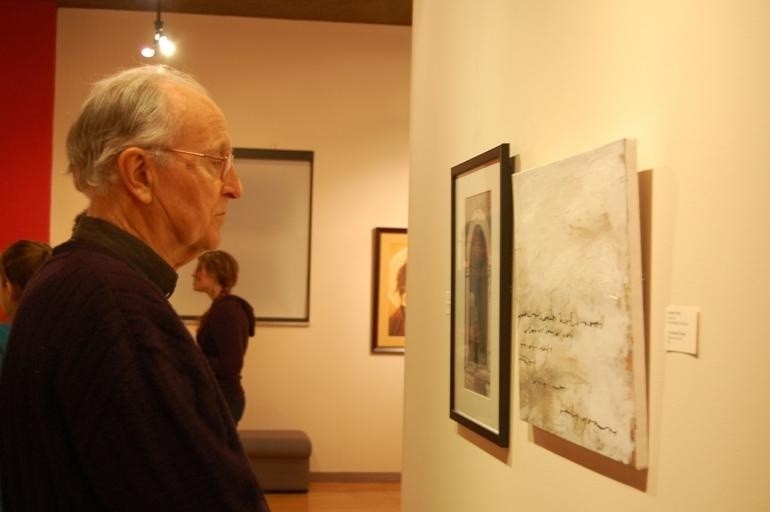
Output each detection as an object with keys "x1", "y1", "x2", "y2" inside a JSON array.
[{"x1": 110, "y1": 145, "x2": 238, "y2": 183}]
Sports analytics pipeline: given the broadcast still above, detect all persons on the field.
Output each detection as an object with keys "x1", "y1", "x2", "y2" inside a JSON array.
[
  {"x1": 0, "y1": 239, "x2": 53, "y2": 359},
  {"x1": 0, "y1": 64, "x2": 272, "y2": 512},
  {"x1": 192, "y1": 250, "x2": 258, "y2": 428}
]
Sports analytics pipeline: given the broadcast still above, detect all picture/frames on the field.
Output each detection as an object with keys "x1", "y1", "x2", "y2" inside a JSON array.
[
  {"x1": 450, "y1": 140, "x2": 512, "y2": 448},
  {"x1": 371, "y1": 227, "x2": 409, "y2": 356}
]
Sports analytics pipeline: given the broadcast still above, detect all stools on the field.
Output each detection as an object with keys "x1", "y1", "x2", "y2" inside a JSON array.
[{"x1": 236, "y1": 429, "x2": 313, "y2": 495}]
